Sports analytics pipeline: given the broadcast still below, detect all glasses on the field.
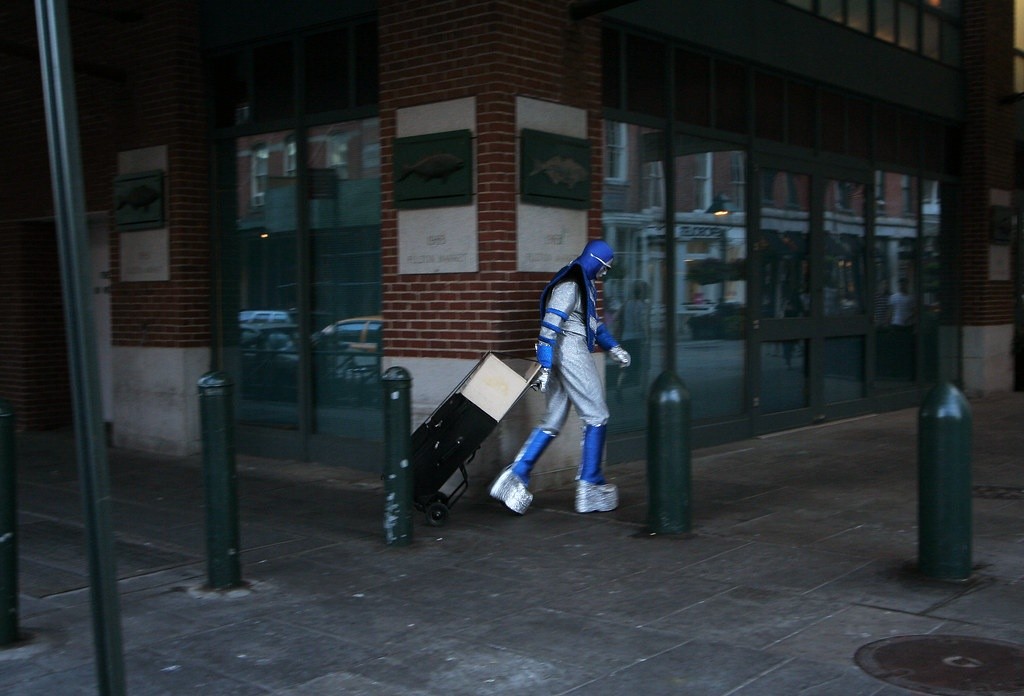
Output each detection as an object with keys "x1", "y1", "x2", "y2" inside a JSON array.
[{"x1": 591, "y1": 254, "x2": 611, "y2": 271}]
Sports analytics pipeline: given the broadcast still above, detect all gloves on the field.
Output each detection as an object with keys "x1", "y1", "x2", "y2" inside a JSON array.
[
  {"x1": 532, "y1": 368, "x2": 551, "y2": 393},
  {"x1": 610, "y1": 345, "x2": 632, "y2": 368}
]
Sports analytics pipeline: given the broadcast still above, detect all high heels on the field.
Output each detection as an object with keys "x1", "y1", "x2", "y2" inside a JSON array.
[
  {"x1": 577, "y1": 479, "x2": 619, "y2": 514},
  {"x1": 491, "y1": 469, "x2": 534, "y2": 515}
]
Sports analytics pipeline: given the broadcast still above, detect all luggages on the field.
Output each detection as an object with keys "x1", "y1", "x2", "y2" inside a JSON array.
[{"x1": 409, "y1": 350, "x2": 544, "y2": 529}]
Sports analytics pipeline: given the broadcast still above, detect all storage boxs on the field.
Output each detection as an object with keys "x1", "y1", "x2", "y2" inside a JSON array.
[{"x1": 460, "y1": 353, "x2": 529, "y2": 422}]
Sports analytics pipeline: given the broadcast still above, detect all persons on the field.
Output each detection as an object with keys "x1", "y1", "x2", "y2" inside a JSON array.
[
  {"x1": 611, "y1": 280, "x2": 652, "y2": 403},
  {"x1": 487, "y1": 240, "x2": 631, "y2": 516},
  {"x1": 776, "y1": 257, "x2": 921, "y2": 378}
]
